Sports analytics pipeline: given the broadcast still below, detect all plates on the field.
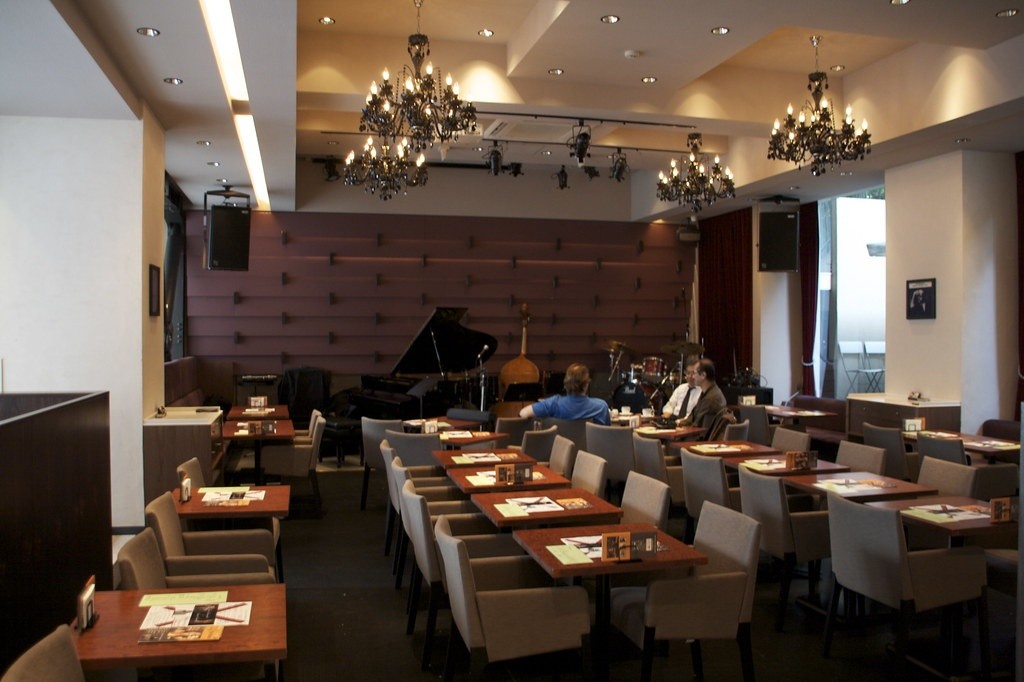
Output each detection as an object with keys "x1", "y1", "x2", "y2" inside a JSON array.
[
  {"x1": 619, "y1": 412, "x2": 633, "y2": 416},
  {"x1": 641, "y1": 415, "x2": 654, "y2": 419}
]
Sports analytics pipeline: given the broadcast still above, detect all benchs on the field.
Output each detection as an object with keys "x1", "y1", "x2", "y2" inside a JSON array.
[
  {"x1": 781, "y1": 395, "x2": 846, "y2": 434},
  {"x1": 165, "y1": 356, "x2": 204, "y2": 406}
]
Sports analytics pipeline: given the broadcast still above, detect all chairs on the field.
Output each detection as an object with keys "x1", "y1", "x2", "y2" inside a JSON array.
[
  {"x1": 838, "y1": 341, "x2": 882, "y2": 399},
  {"x1": 0, "y1": 396, "x2": 1021, "y2": 682},
  {"x1": 863, "y1": 341, "x2": 885, "y2": 392},
  {"x1": 279, "y1": 367, "x2": 328, "y2": 427}
]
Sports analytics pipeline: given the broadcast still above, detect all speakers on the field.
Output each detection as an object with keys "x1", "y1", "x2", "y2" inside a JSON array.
[
  {"x1": 203, "y1": 204, "x2": 250, "y2": 271},
  {"x1": 758, "y1": 211, "x2": 800, "y2": 273}
]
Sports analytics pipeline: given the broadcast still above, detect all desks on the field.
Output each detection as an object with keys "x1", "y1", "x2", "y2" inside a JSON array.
[
  {"x1": 68, "y1": 583, "x2": 287, "y2": 682},
  {"x1": 864, "y1": 495, "x2": 1019, "y2": 682},
  {"x1": 174, "y1": 485, "x2": 291, "y2": 584},
  {"x1": 437, "y1": 429, "x2": 511, "y2": 450},
  {"x1": 612, "y1": 412, "x2": 662, "y2": 426},
  {"x1": 769, "y1": 407, "x2": 838, "y2": 433},
  {"x1": 445, "y1": 464, "x2": 572, "y2": 495},
  {"x1": 431, "y1": 448, "x2": 538, "y2": 471},
  {"x1": 961, "y1": 435, "x2": 1020, "y2": 466},
  {"x1": 236, "y1": 374, "x2": 279, "y2": 396},
  {"x1": 635, "y1": 423, "x2": 709, "y2": 457},
  {"x1": 470, "y1": 487, "x2": 625, "y2": 587},
  {"x1": 670, "y1": 441, "x2": 783, "y2": 488},
  {"x1": 901, "y1": 428, "x2": 965, "y2": 445},
  {"x1": 781, "y1": 471, "x2": 939, "y2": 628},
  {"x1": 222, "y1": 420, "x2": 296, "y2": 486},
  {"x1": 848, "y1": 397, "x2": 961, "y2": 452},
  {"x1": 225, "y1": 405, "x2": 289, "y2": 421},
  {"x1": 722, "y1": 454, "x2": 851, "y2": 585},
  {"x1": 512, "y1": 522, "x2": 710, "y2": 682},
  {"x1": 403, "y1": 416, "x2": 481, "y2": 431}
]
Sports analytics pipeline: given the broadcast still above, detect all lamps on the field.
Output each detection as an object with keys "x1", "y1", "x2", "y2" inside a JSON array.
[
  {"x1": 486, "y1": 145, "x2": 504, "y2": 177},
  {"x1": 656, "y1": 127, "x2": 737, "y2": 221},
  {"x1": 324, "y1": 161, "x2": 341, "y2": 183},
  {"x1": 343, "y1": 135, "x2": 429, "y2": 202},
  {"x1": 567, "y1": 124, "x2": 593, "y2": 168},
  {"x1": 767, "y1": 34, "x2": 872, "y2": 177},
  {"x1": 609, "y1": 151, "x2": 628, "y2": 183},
  {"x1": 556, "y1": 165, "x2": 570, "y2": 190},
  {"x1": 358, "y1": 0, "x2": 478, "y2": 153}
]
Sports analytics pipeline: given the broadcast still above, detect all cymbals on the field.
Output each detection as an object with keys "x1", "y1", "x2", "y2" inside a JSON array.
[
  {"x1": 661, "y1": 341, "x2": 705, "y2": 356},
  {"x1": 596, "y1": 339, "x2": 627, "y2": 353}
]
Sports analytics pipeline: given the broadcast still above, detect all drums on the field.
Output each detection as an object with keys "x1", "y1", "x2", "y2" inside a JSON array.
[
  {"x1": 626, "y1": 362, "x2": 662, "y2": 412},
  {"x1": 642, "y1": 357, "x2": 667, "y2": 382},
  {"x1": 539, "y1": 368, "x2": 568, "y2": 399},
  {"x1": 663, "y1": 368, "x2": 686, "y2": 385}
]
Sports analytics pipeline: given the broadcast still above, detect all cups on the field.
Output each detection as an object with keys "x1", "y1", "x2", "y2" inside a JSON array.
[
  {"x1": 622, "y1": 406, "x2": 631, "y2": 413},
  {"x1": 642, "y1": 409, "x2": 652, "y2": 416},
  {"x1": 611, "y1": 409, "x2": 618, "y2": 418}
]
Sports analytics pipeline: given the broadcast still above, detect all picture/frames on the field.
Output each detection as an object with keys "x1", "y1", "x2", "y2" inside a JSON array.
[
  {"x1": 906, "y1": 277, "x2": 937, "y2": 320},
  {"x1": 148, "y1": 263, "x2": 161, "y2": 317}
]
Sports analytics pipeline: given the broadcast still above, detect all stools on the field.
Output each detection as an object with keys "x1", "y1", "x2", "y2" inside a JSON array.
[{"x1": 319, "y1": 414, "x2": 365, "y2": 469}]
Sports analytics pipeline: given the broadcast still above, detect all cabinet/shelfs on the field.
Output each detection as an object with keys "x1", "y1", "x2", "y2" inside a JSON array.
[{"x1": 142, "y1": 406, "x2": 224, "y2": 505}]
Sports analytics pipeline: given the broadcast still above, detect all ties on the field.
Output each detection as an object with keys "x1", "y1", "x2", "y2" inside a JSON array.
[{"x1": 679, "y1": 386, "x2": 696, "y2": 418}]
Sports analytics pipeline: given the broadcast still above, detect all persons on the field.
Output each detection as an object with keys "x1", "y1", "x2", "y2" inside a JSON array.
[
  {"x1": 518, "y1": 363, "x2": 612, "y2": 425},
  {"x1": 677, "y1": 360, "x2": 728, "y2": 441},
  {"x1": 661, "y1": 363, "x2": 704, "y2": 421},
  {"x1": 909, "y1": 289, "x2": 930, "y2": 314}
]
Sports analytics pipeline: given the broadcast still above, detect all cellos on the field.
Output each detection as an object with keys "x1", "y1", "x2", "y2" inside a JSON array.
[{"x1": 490, "y1": 303, "x2": 540, "y2": 424}]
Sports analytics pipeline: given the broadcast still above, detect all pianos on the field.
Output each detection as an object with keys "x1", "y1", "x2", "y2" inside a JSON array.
[{"x1": 358, "y1": 306, "x2": 498, "y2": 428}]
